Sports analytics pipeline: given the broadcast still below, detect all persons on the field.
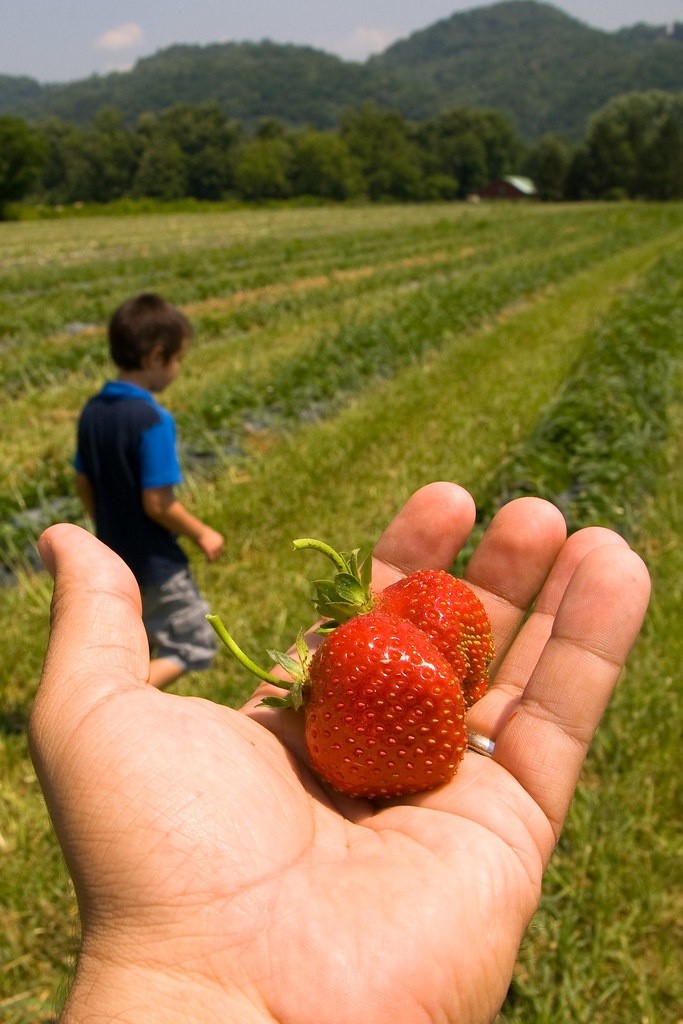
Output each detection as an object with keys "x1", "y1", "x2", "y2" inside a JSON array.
[
  {"x1": 27, "y1": 481, "x2": 652, "y2": 1024},
  {"x1": 74, "y1": 293, "x2": 225, "y2": 690}
]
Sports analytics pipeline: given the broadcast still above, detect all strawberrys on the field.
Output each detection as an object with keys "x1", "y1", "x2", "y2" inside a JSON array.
[{"x1": 295, "y1": 568, "x2": 495, "y2": 799}]
